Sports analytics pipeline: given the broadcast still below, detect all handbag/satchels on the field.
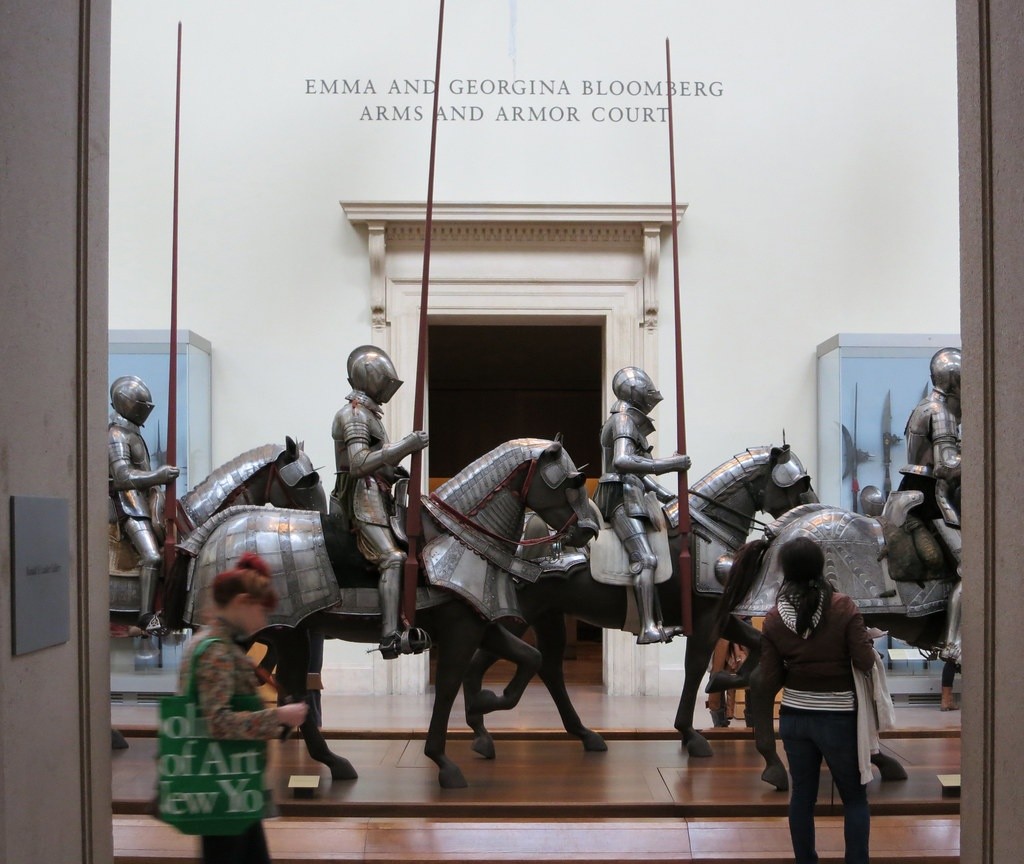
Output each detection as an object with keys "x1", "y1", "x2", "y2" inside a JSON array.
[{"x1": 157, "y1": 690, "x2": 271, "y2": 839}]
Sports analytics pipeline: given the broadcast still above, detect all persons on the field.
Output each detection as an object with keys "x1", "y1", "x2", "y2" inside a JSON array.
[
  {"x1": 104, "y1": 376, "x2": 181, "y2": 633},
  {"x1": 179, "y1": 556, "x2": 308, "y2": 864},
  {"x1": 591, "y1": 366, "x2": 691, "y2": 645},
  {"x1": 331, "y1": 344, "x2": 431, "y2": 660},
  {"x1": 895, "y1": 347, "x2": 961, "y2": 666},
  {"x1": 760, "y1": 537, "x2": 876, "y2": 864},
  {"x1": 940, "y1": 659, "x2": 960, "y2": 710}
]
